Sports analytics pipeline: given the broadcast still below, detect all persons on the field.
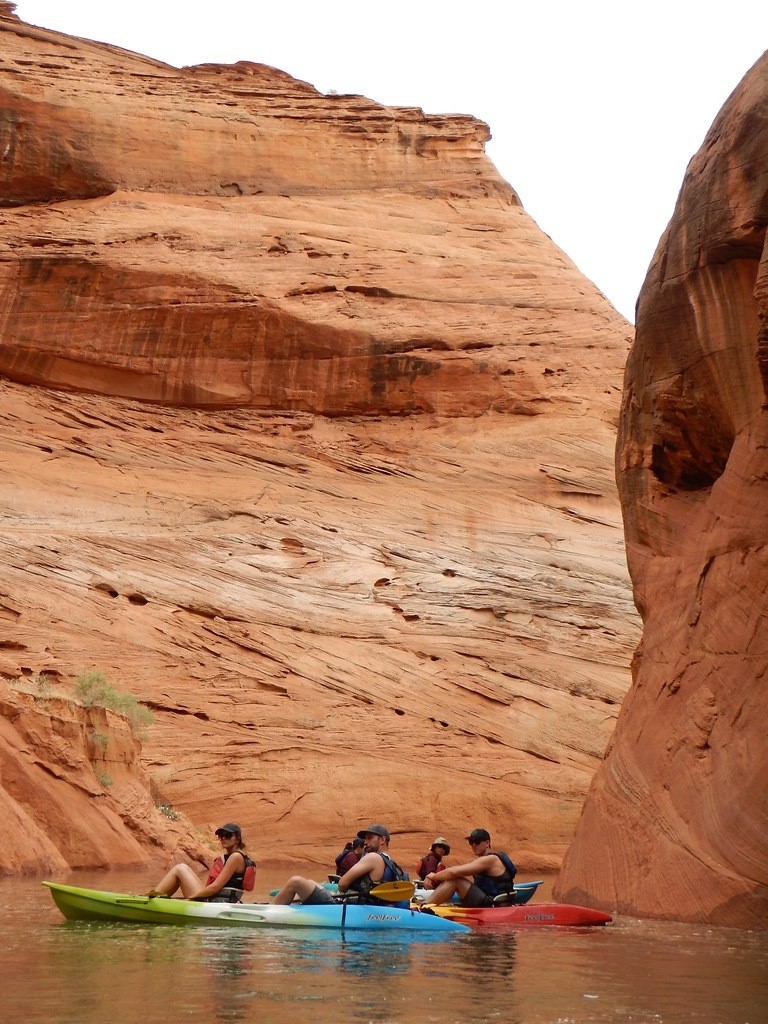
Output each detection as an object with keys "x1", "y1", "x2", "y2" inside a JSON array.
[
  {"x1": 421, "y1": 837, "x2": 450, "y2": 881},
  {"x1": 336, "y1": 838, "x2": 365, "y2": 884},
  {"x1": 153, "y1": 823, "x2": 248, "y2": 903},
  {"x1": 270, "y1": 825, "x2": 397, "y2": 907},
  {"x1": 422, "y1": 829, "x2": 513, "y2": 908}
]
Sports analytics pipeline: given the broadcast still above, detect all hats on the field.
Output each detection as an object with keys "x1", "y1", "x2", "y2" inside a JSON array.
[
  {"x1": 215, "y1": 822, "x2": 241, "y2": 837},
  {"x1": 429, "y1": 837, "x2": 450, "y2": 855},
  {"x1": 357, "y1": 824, "x2": 390, "y2": 841},
  {"x1": 465, "y1": 829, "x2": 490, "y2": 841}
]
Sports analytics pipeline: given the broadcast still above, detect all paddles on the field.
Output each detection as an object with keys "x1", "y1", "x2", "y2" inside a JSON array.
[{"x1": 260, "y1": 880, "x2": 416, "y2": 903}]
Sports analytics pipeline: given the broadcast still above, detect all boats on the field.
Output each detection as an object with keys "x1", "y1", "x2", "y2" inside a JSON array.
[
  {"x1": 41, "y1": 879, "x2": 474, "y2": 934},
  {"x1": 272, "y1": 879, "x2": 546, "y2": 906},
  {"x1": 414, "y1": 902, "x2": 615, "y2": 927}
]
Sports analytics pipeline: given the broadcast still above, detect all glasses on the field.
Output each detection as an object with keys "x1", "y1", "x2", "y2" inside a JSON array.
[
  {"x1": 437, "y1": 844, "x2": 447, "y2": 850},
  {"x1": 468, "y1": 839, "x2": 486, "y2": 845},
  {"x1": 218, "y1": 832, "x2": 235, "y2": 840}
]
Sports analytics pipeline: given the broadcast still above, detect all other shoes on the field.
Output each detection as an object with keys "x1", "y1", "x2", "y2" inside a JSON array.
[{"x1": 411, "y1": 896, "x2": 427, "y2": 907}]
